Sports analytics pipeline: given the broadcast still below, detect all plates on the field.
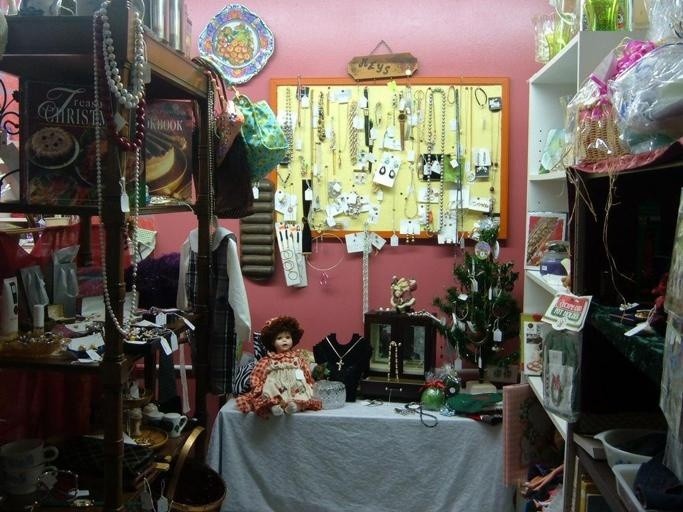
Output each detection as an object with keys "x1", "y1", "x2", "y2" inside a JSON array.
[
  {"x1": 195, "y1": 2, "x2": 276, "y2": 86},
  {"x1": 24, "y1": 127, "x2": 80, "y2": 171}
]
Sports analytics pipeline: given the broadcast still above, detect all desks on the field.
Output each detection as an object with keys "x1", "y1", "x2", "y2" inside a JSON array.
[{"x1": 208, "y1": 383, "x2": 517, "y2": 511}]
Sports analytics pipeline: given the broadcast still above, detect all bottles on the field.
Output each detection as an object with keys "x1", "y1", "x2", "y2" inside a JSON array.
[
  {"x1": 531, "y1": 0, "x2": 620, "y2": 64},
  {"x1": 540, "y1": 240, "x2": 569, "y2": 288}
]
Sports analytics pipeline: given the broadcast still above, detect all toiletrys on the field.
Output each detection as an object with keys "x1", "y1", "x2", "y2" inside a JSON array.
[
  {"x1": 1, "y1": 276, "x2": 19, "y2": 342},
  {"x1": 3, "y1": 276, "x2": 19, "y2": 339},
  {"x1": 34, "y1": 303, "x2": 44, "y2": 329},
  {"x1": 33, "y1": 303, "x2": 44, "y2": 337},
  {"x1": 422, "y1": 382, "x2": 442, "y2": 409}
]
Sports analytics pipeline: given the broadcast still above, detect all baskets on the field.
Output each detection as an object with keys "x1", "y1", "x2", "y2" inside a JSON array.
[{"x1": 575, "y1": 102, "x2": 632, "y2": 162}]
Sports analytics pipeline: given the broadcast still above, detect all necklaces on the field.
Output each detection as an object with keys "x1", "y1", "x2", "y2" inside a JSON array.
[
  {"x1": 324, "y1": 336, "x2": 364, "y2": 372},
  {"x1": 387, "y1": 340, "x2": 399, "y2": 383},
  {"x1": 275, "y1": 76, "x2": 500, "y2": 244},
  {"x1": 90, "y1": 0, "x2": 147, "y2": 337}
]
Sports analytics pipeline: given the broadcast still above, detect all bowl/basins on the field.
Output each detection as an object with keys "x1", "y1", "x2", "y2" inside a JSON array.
[
  {"x1": 75, "y1": 129, "x2": 188, "y2": 194},
  {"x1": 592, "y1": 428, "x2": 653, "y2": 472},
  {"x1": 123, "y1": 386, "x2": 154, "y2": 408},
  {"x1": 126, "y1": 426, "x2": 170, "y2": 450},
  {"x1": 43, "y1": 216, "x2": 72, "y2": 227}
]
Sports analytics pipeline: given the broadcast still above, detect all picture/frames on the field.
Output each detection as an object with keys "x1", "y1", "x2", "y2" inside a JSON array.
[{"x1": 271, "y1": 74, "x2": 510, "y2": 244}]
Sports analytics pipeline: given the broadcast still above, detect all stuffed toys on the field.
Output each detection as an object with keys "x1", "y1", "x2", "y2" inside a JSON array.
[{"x1": 235, "y1": 314, "x2": 322, "y2": 422}]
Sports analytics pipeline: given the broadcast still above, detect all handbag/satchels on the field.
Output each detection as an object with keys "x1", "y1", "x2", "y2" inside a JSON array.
[
  {"x1": 188, "y1": 56, "x2": 257, "y2": 222},
  {"x1": 202, "y1": 56, "x2": 290, "y2": 182},
  {"x1": 194, "y1": 57, "x2": 247, "y2": 167}
]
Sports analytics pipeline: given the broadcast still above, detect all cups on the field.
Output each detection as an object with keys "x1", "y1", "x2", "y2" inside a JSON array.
[
  {"x1": 145, "y1": 411, "x2": 165, "y2": 431},
  {"x1": 162, "y1": 412, "x2": 187, "y2": 438},
  {"x1": 1, "y1": 438, "x2": 61, "y2": 471},
  {"x1": 0, "y1": 465, "x2": 58, "y2": 496}
]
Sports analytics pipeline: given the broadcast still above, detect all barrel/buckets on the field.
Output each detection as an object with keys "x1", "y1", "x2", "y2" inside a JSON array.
[{"x1": 150, "y1": 426, "x2": 227, "y2": 511}]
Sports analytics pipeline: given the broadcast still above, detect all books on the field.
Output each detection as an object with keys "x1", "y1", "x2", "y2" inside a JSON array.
[{"x1": 571, "y1": 455, "x2": 612, "y2": 512}]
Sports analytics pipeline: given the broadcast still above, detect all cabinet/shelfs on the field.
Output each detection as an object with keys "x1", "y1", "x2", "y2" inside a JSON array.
[
  {"x1": 565, "y1": 159, "x2": 679, "y2": 509},
  {"x1": 0, "y1": 10, "x2": 219, "y2": 512},
  {"x1": 517, "y1": 29, "x2": 635, "y2": 511}
]
